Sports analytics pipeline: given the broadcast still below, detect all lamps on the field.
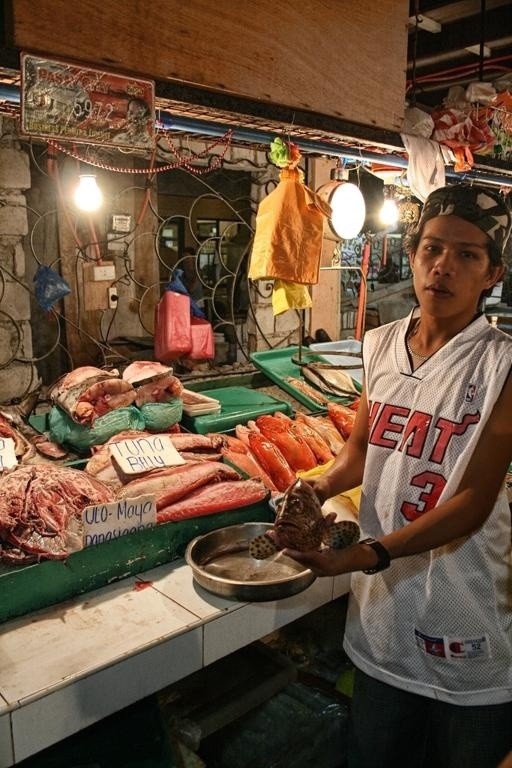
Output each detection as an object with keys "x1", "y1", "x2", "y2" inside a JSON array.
[
  {"x1": 316, "y1": 167, "x2": 367, "y2": 242},
  {"x1": 73, "y1": 159, "x2": 103, "y2": 213},
  {"x1": 379, "y1": 187, "x2": 400, "y2": 226}
]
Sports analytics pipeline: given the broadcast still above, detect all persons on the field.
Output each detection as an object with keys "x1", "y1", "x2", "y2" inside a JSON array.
[
  {"x1": 181, "y1": 246, "x2": 206, "y2": 312},
  {"x1": 265, "y1": 181, "x2": 512, "y2": 768}
]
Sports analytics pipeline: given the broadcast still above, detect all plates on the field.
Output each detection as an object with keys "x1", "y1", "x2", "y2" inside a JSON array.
[{"x1": 187, "y1": 520, "x2": 318, "y2": 602}]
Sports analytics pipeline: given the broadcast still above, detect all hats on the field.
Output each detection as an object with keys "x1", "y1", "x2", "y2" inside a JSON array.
[{"x1": 417, "y1": 184, "x2": 512, "y2": 255}]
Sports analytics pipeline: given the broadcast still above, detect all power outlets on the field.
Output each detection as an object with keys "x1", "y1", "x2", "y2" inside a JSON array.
[
  {"x1": 107, "y1": 287, "x2": 118, "y2": 310},
  {"x1": 93, "y1": 265, "x2": 116, "y2": 281}
]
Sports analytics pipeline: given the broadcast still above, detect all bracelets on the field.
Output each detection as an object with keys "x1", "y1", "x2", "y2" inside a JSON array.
[{"x1": 357, "y1": 537, "x2": 392, "y2": 576}]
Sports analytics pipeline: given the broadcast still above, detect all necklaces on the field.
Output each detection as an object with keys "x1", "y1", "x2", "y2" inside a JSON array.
[{"x1": 405, "y1": 326, "x2": 430, "y2": 362}]
